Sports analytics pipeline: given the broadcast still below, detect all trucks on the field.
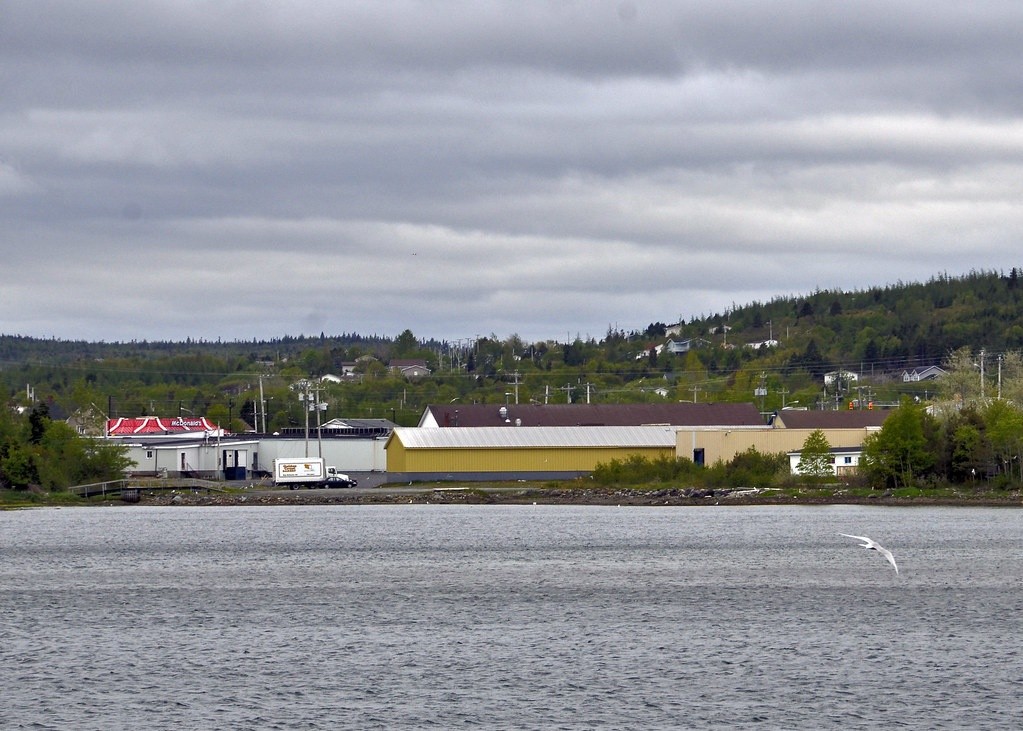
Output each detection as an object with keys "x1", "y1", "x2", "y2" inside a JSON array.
[{"x1": 272, "y1": 458, "x2": 349, "y2": 491}]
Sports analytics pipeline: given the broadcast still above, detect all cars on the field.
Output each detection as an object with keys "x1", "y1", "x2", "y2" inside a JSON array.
[{"x1": 317, "y1": 476, "x2": 358, "y2": 489}]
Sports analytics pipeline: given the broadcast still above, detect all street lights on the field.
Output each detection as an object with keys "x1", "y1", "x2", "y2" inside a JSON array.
[
  {"x1": 505, "y1": 392, "x2": 515, "y2": 423},
  {"x1": 974, "y1": 363, "x2": 984, "y2": 400}
]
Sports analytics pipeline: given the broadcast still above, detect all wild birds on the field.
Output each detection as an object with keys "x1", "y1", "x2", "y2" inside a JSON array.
[{"x1": 837, "y1": 530, "x2": 901, "y2": 576}]
